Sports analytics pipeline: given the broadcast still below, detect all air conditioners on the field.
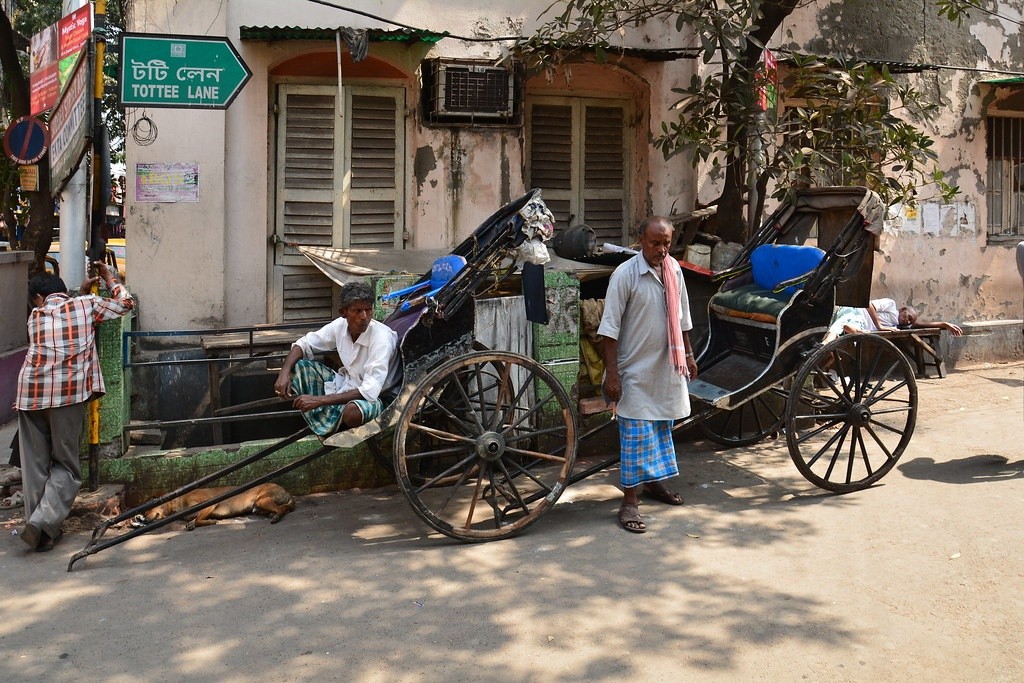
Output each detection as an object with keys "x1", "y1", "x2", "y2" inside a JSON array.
[{"x1": 431, "y1": 62, "x2": 514, "y2": 116}]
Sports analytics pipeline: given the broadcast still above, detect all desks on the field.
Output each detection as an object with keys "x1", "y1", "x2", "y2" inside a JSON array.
[{"x1": 201, "y1": 325, "x2": 322, "y2": 447}]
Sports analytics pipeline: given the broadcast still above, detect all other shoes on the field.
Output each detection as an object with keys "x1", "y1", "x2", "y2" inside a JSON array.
[
  {"x1": 36, "y1": 531, "x2": 63, "y2": 551},
  {"x1": 20, "y1": 522, "x2": 39, "y2": 550}
]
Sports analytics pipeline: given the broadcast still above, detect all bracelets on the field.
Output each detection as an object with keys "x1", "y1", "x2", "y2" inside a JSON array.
[{"x1": 686, "y1": 352, "x2": 694, "y2": 358}]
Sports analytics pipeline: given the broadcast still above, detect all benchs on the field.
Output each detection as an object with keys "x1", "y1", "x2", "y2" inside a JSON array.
[
  {"x1": 387, "y1": 254, "x2": 469, "y2": 351},
  {"x1": 839, "y1": 329, "x2": 946, "y2": 382},
  {"x1": 710, "y1": 243, "x2": 828, "y2": 330}
]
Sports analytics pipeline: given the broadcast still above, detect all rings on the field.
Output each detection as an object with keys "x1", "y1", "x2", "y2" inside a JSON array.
[{"x1": 275, "y1": 390, "x2": 281, "y2": 395}]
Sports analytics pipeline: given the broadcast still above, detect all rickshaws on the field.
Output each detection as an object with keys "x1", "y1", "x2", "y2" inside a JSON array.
[
  {"x1": 481, "y1": 185, "x2": 918, "y2": 517},
  {"x1": 66, "y1": 187, "x2": 581, "y2": 575}
]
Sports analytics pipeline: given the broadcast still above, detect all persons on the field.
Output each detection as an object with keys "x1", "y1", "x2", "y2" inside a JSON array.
[
  {"x1": 274, "y1": 280, "x2": 403, "y2": 436},
  {"x1": 15, "y1": 259, "x2": 134, "y2": 553},
  {"x1": 813, "y1": 297, "x2": 963, "y2": 388},
  {"x1": 596, "y1": 215, "x2": 697, "y2": 533}
]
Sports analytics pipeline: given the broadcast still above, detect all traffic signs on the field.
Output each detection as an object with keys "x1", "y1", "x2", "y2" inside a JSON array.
[{"x1": 116, "y1": 32, "x2": 253, "y2": 111}]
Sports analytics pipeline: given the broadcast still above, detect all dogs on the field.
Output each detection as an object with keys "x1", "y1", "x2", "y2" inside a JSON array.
[{"x1": 144, "y1": 482, "x2": 296, "y2": 531}]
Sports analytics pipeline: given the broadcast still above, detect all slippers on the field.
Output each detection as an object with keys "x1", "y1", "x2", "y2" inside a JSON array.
[
  {"x1": 618, "y1": 506, "x2": 646, "y2": 532},
  {"x1": 642, "y1": 482, "x2": 684, "y2": 505}
]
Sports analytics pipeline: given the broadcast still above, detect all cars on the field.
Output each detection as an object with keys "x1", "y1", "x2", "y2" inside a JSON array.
[
  {"x1": 105, "y1": 238, "x2": 125, "y2": 277},
  {"x1": 45, "y1": 242, "x2": 61, "y2": 275}
]
